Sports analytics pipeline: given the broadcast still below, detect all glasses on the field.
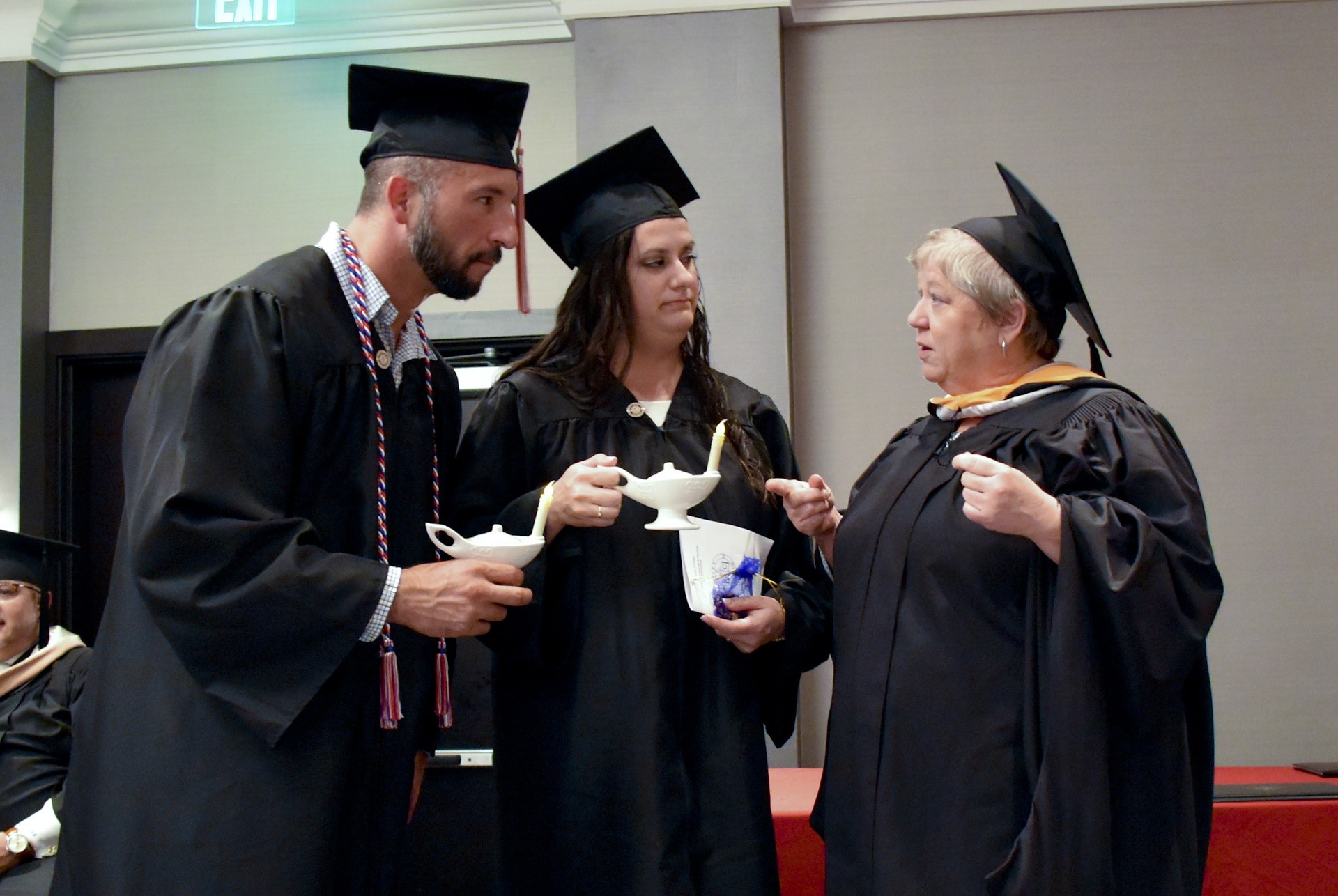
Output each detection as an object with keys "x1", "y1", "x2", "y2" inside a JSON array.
[{"x1": 0, "y1": 582, "x2": 41, "y2": 599}]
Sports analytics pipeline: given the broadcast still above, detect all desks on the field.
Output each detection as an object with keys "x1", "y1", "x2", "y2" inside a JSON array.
[{"x1": 767, "y1": 757, "x2": 1338, "y2": 896}]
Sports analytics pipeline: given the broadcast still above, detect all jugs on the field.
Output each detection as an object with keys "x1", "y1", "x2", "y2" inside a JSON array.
[
  {"x1": 597, "y1": 461, "x2": 722, "y2": 530},
  {"x1": 424, "y1": 522, "x2": 546, "y2": 569}
]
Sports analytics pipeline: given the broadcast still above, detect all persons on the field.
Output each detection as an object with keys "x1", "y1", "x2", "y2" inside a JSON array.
[
  {"x1": 56, "y1": 65, "x2": 535, "y2": 895},
  {"x1": 0, "y1": 527, "x2": 93, "y2": 896},
  {"x1": 449, "y1": 125, "x2": 834, "y2": 896},
  {"x1": 764, "y1": 159, "x2": 1224, "y2": 896}
]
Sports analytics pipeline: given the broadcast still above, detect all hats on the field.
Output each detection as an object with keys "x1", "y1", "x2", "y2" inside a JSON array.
[
  {"x1": 525, "y1": 127, "x2": 699, "y2": 269},
  {"x1": 348, "y1": 63, "x2": 532, "y2": 315},
  {"x1": 0, "y1": 529, "x2": 79, "y2": 650},
  {"x1": 956, "y1": 164, "x2": 1110, "y2": 375}
]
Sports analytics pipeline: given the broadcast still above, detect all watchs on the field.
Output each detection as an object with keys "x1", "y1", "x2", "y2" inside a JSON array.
[{"x1": 6, "y1": 826, "x2": 36, "y2": 862}]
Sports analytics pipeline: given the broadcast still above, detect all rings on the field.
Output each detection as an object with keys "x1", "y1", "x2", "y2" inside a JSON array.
[
  {"x1": 597, "y1": 506, "x2": 602, "y2": 519},
  {"x1": 726, "y1": 637, "x2": 731, "y2": 643}
]
